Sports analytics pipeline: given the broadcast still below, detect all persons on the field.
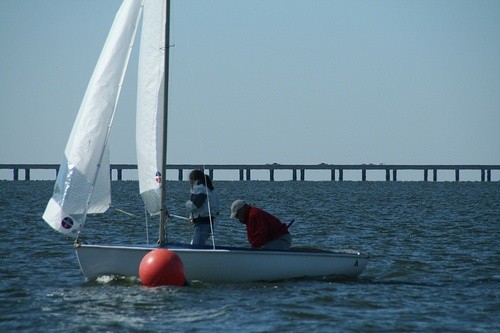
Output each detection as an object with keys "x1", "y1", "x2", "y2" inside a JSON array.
[
  {"x1": 230, "y1": 200, "x2": 292, "y2": 252},
  {"x1": 185, "y1": 169, "x2": 216, "y2": 246}
]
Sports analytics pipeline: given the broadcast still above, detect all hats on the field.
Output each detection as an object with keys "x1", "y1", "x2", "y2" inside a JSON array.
[{"x1": 230, "y1": 200, "x2": 245, "y2": 219}]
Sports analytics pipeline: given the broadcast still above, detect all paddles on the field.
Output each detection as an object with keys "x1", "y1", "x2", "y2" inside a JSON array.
[{"x1": 287, "y1": 219, "x2": 295, "y2": 228}]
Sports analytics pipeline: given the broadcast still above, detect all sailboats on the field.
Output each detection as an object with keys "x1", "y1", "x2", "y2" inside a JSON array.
[{"x1": 40, "y1": 0, "x2": 371, "y2": 282}]
